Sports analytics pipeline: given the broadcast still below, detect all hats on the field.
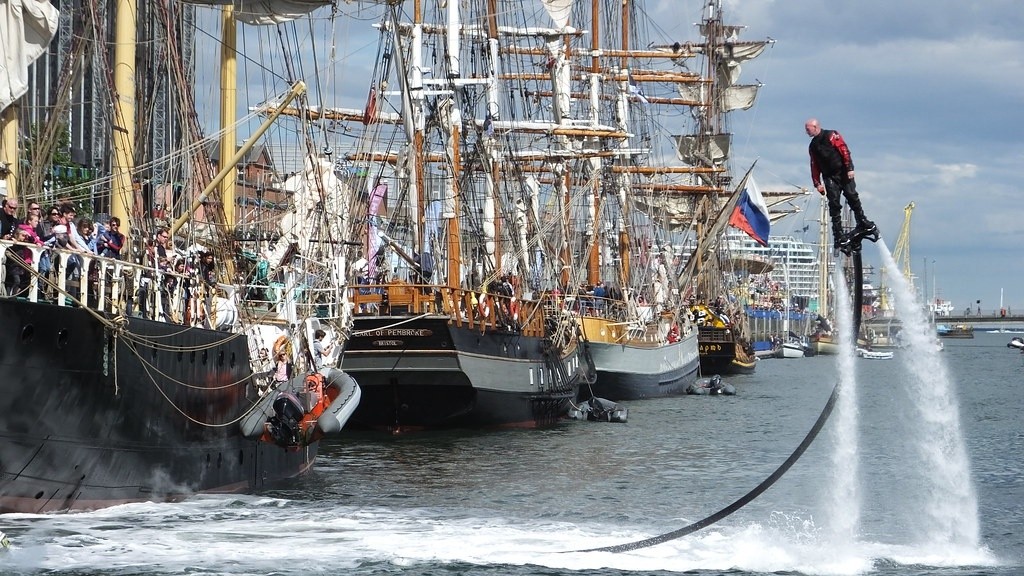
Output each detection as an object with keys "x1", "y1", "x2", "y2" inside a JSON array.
[{"x1": 52, "y1": 225, "x2": 67, "y2": 234}]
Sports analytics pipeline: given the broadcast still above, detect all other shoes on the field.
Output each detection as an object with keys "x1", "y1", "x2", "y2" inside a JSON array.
[
  {"x1": 834, "y1": 232, "x2": 848, "y2": 244},
  {"x1": 857, "y1": 218, "x2": 874, "y2": 229}
]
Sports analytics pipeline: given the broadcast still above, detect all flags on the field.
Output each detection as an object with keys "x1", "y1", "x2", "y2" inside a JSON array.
[{"x1": 727, "y1": 188, "x2": 770, "y2": 247}]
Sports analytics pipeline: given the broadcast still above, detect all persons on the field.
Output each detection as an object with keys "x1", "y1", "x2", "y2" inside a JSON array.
[
  {"x1": 462, "y1": 271, "x2": 640, "y2": 339},
  {"x1": 1, "y1": 192, "x2": 302, "y2": 333},
  {"x1": 345, "y1": 248, "x2": 446, "y2": 319},
  {"x1": 273, "y1": 330, "x2": 335, "y2": 442},
  {"x1": 662, "y1": 275, "x2": 831, "y2": 346},
  {"x1": 806, "y1": 116, "x2": 877, "y2": 245}
]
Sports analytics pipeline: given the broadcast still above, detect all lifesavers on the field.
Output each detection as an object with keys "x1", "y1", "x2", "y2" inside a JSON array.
[
  {"x1": 509, "y1": 296, "x2": 519, "y2": 321},
  {"x1": 273, "y1": 335, "x2": 293, "y2": 362},
  {"x1": 479, "y1": 293, "x2": 490, "y2": 317}
]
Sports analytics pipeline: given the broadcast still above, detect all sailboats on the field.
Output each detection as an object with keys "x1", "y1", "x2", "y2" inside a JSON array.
[{"x1": 0, "y1": 1, "x2": 974, "y2": 515}]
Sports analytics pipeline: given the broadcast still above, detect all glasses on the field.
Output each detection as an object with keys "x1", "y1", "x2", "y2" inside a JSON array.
[
  {"x1": 29, "y1": 208, "x2": 40, "y2": 210},
  {"x1": 160, "y1": 235, "x2": 168, "y2": 238},
  {"x1": 6, "y1": 204, "x2": 16, "y2": 210},
  {"x1": 50, "y1": 213, "x2": 60, "y2": 216},
  {"x1": 111, "y1": 223, "x2": 118, "y2": 226}
]
